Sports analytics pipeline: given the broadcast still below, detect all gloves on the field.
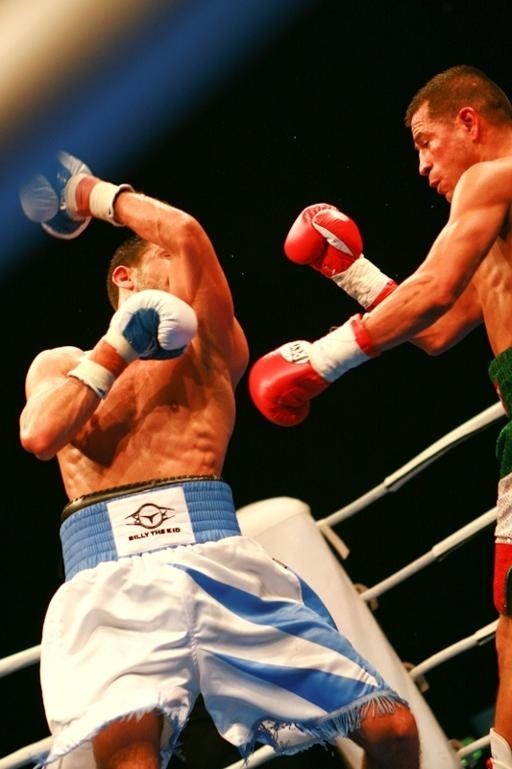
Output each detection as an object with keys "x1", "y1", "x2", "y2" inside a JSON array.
[
  {"x1": 17, "y1": 149, "x2": 134, "y2": 239},
  {"x1": 284, "y1": 203, "x2": 397, "y2": 312},
  {"x1": 66, "y1": 288, "x2": 198, "y2": 400},
  {"x1": 248, "y1": 314, "x2": 380, "y2": 427}
]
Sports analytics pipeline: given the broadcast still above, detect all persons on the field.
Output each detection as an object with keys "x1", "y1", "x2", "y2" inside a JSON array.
[
  {"x1": 245, "y1": 62, "x2": 512, "y2": 769},
  {"x1": 12, "y1": 146, "x2": 424, "y2": 769}
]
